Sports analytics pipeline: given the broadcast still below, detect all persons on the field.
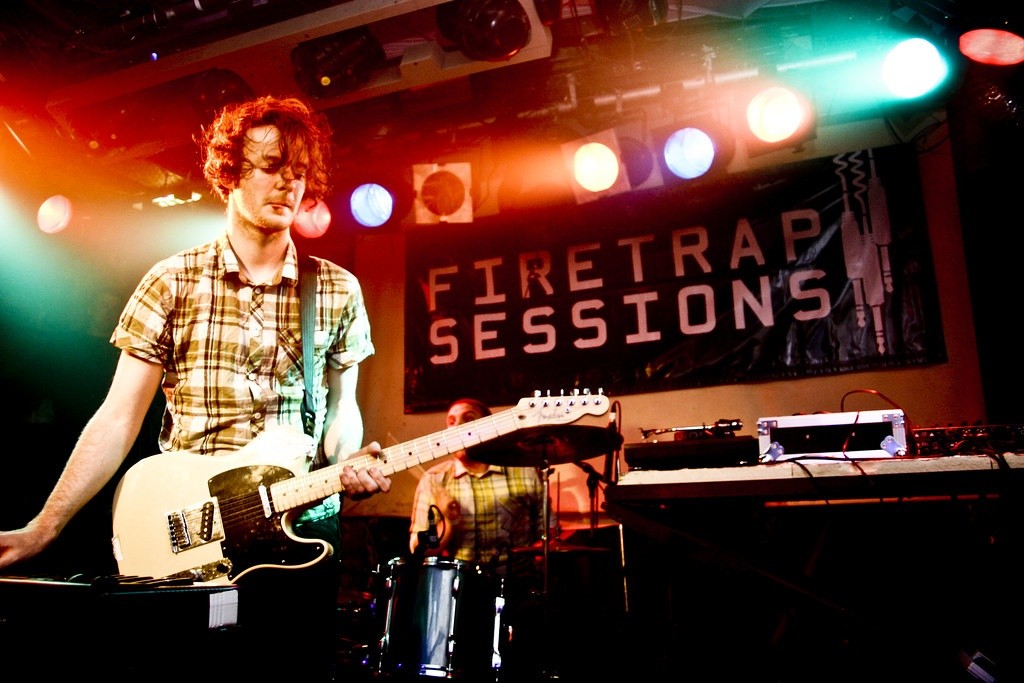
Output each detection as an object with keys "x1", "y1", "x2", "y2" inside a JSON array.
[
  {"x1": 0, "y1": 98, "x2": 395, "y2": 569},
  {"x1": 410, "y1": 399, "x2": 561, "y2": 667}
]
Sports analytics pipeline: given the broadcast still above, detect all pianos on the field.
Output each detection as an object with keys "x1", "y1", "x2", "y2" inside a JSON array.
[{"x1": 609, "y1": 453, "x2": 1024, "y2": 512}]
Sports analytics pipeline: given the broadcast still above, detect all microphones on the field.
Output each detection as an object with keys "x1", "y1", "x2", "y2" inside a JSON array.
[
  {"x1": 429, "y1": 509, "x2": 440, "y2": 549},
  {"x1": 602, "y1": 404, "x2": 616, "y2": 482}
]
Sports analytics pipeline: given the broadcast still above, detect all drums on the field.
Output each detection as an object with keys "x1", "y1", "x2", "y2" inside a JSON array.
[{"x1": 375, "y1": 559, "x2": 506, "y2": 683}]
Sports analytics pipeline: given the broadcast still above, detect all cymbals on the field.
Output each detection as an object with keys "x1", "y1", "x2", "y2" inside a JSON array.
[
  {"x1": 470, "y1": 424, "x2": 627, "y2": 466},
  {"x1": 504, "y1": 542, "x2": 609, "y2": 556}
]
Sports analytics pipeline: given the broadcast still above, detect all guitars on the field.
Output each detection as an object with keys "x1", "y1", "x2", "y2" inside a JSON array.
[{"x1": 112, "y1": 387, "x2": 609, "y2": 583}]
